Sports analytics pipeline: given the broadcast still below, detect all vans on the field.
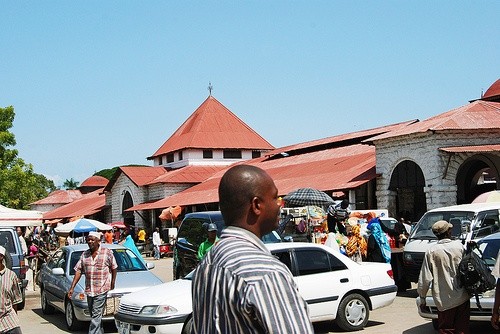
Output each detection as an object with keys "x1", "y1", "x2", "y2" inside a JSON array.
[
  {"x1": 0, "y1": 226, "x2": 29, "y2": 309},
  {"x1": 402, "y1": 202, "x2": 500, "y2": 280},
  {"x1": 172, "y1": 211, "x2": 282, "y2": 281}
]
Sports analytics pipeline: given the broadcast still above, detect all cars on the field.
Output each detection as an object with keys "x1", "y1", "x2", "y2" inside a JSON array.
[
  {"x1": 416, "y1": 234, "x2": 500, "y2": 327},
  {"x1": 358, "y1": 217, "x2": 412, "y2": 253},
  {"x1": 35, "y1": 243, "x2": 164, "y2": 330},
  {"x1": 114, "y1": 242, "x2": 397, "y2": 334}
]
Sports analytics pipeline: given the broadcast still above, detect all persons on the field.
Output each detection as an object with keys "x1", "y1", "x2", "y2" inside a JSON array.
[
  {"x1": 68, "y1": 231, "x2": 118, "y2": 334},
  {"x1": 417, "y1": 220, "x2": 474, "y2": 334},
  {"x1": 197, "y1": 223, "x2": 220, "y2": 261},
  {"x1": 394, "y1": 218, "x2": 407, "y2": 248},
  {"x1": 138, "y1": 228, "x2": 146, "y2": 243},
  {"x1": 327, "y1": 199, "x2": 349, "y2": 235},
  {"x1": 16, "y1": 226, "x2": 28, "y2": 257},
  {"x1": 490, "y1": 248, "x2": 500, "y2": 329},
  {"x1": 153, "y1": 227, "x2": 161, "y2": 260},
  {"x1": 28, "y1": 224, "x2": 135, "y2": 283},
  {"x1": 346, "y1": 225, "x2": 367, "y2": 262},
  {"x1": 183, "y1": 164, "x2": 318, "y2": 334},
  {"x1": 366, "y1": 212, "x2": 391, "y2": 263},
  {"x1": 0, "y1": 245, "x2": 23, "y2": 334}
]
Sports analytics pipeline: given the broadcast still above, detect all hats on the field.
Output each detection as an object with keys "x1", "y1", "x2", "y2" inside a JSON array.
[
  {"x1": 0, "y1": 246, "x2": 7, "y2": 256},
  {"x1": 207, "y1": 223, "x2": 218, "y2": 232},
  {"x1": 88, "y1": 231, "x2": 101, "y2": 240},
  {"x1": 431, "y1": 220, "x2": 453, "y2": 234}
]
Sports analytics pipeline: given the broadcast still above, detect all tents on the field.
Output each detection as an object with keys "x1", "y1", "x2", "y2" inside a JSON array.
[{"x1": 0, "y1": 204, "x2": 43, "y2": 291}]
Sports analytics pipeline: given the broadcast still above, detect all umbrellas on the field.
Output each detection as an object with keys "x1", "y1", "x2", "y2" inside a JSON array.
[
  {"x1": 299, "y1": 205, "x2": 326, "y2": 220},
  {"x1": 54, "y1": 218, "x2": 112, "y2": 244},
  {"x1": 282, "y1": 187, "x2": 337, "y2": 219}
]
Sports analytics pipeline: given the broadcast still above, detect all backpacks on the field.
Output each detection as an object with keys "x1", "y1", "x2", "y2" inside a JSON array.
[{"x1": 457, "y1": 242, "x2": 495, "y2": 310}]
